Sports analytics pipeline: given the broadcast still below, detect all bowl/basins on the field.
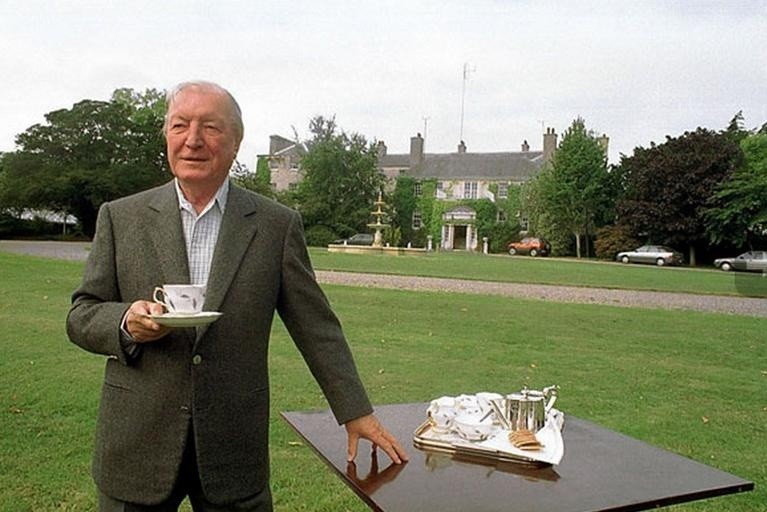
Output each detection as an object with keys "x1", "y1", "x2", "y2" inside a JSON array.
[{"x1": 455, "y1": 412, "x2": 494, "y2": 442}]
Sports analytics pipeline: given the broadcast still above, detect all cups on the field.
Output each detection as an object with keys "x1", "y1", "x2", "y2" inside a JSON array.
[
  {"x1": 152, "y1": 283, "x2": 209, "y2": 316},
  {"x1": 425, "y1": 396, "x2": 456, "y2": 435}
]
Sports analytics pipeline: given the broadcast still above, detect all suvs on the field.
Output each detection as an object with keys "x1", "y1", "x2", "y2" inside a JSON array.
[
  {"x1": 335, "y1": 233, "x2": 374, "y2": 245},
  {"x1": 506, "y1": 236, "x2": 551, "y2": 256}
]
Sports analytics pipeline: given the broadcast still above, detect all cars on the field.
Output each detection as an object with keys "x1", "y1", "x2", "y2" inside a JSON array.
[
  {"x1": 714, "y1": 251, "x2": 767, "y2": 273},
  {"x1": 615, "y1": 243, "x2": 684, "y2": 267}
]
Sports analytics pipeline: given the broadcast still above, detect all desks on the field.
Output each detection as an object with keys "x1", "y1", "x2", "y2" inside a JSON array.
[{"x1": 279, "y1": 391, "x2": 754, "y2": 512}]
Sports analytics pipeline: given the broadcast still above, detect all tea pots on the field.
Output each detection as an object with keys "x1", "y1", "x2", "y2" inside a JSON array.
[{"x1": 489, "y1": 383, "x2": 559, "y2": 434}]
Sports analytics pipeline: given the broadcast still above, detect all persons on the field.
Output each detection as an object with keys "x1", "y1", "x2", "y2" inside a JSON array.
[
  {"x1": 346, "y1": 450, "x2": 409, "y2": 496},
  {"x1": 64, "y1": 77, "x2": 409, "y2": 511}
]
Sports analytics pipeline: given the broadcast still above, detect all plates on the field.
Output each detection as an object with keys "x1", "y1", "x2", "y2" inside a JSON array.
[{"x1": 145, "y1": 311, "x2": 225, "y2": 327}]
